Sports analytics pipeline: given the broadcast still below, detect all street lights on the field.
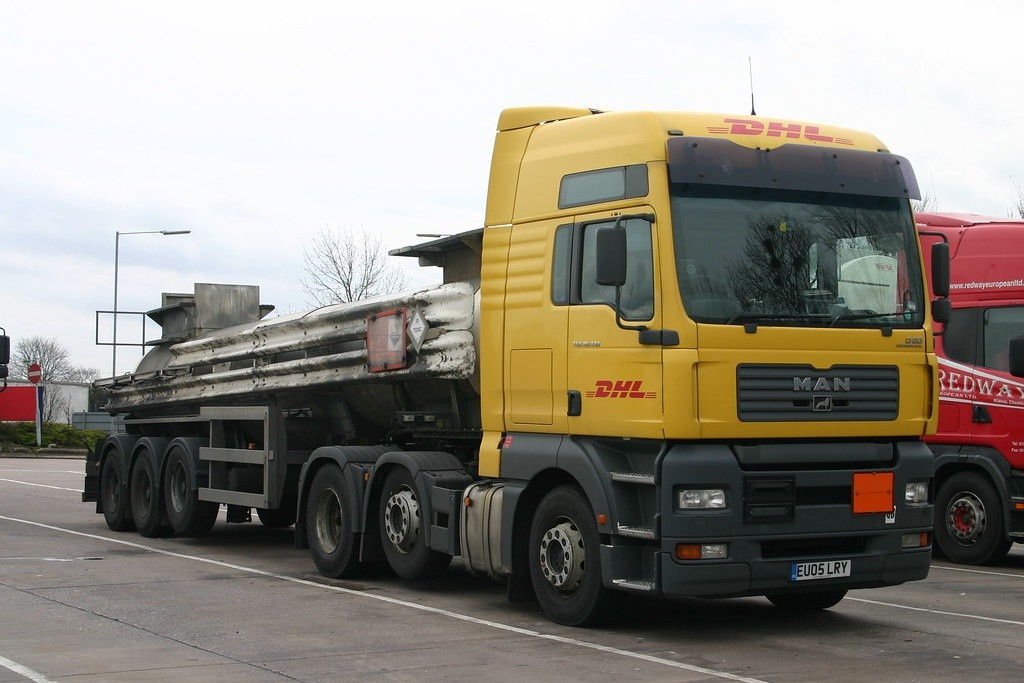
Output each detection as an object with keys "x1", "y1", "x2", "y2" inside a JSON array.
[{"x1": 111, "y1": 228, "x2": 191, "y2": 377}]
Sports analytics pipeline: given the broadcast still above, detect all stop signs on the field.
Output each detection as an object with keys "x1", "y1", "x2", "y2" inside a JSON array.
[{"x1": 28, "y1": 363, "x2": 41, "y2": 384}]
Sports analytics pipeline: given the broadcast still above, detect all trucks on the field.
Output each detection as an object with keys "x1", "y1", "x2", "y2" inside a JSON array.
[{"x1": 811, "y1": 210, "x2": 1024, "y2": 566}]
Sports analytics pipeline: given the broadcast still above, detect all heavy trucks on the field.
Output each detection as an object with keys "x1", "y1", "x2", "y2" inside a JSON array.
[{"x1": 69, "y1": 103, "x2": 953, "y2": 629}]
